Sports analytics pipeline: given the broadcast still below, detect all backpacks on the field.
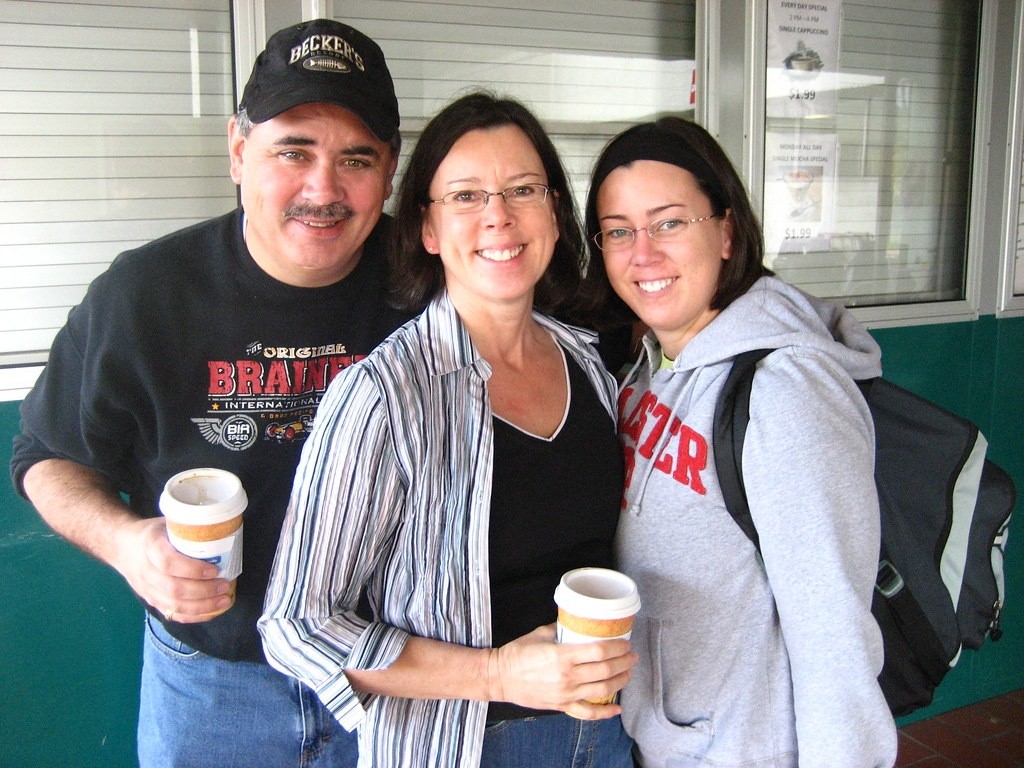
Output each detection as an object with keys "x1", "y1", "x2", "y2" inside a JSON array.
[{"x1": 712, "y1": 345, "x2": 1018, "y2": 717}]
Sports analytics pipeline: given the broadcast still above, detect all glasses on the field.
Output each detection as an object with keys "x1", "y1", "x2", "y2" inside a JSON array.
[
  {"x1": 591, "y1": 210, "x2": 728, "y2": 254},
  {"x1": 424, "y1": 184, "x2": 558, "y2": 214}
]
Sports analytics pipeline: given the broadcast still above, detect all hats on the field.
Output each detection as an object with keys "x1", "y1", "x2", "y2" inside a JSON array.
[{"x1": 240, "y1": 20, "x2": 401, "y2": 145}]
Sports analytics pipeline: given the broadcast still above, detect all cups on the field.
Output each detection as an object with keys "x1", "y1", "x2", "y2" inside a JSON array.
[
  {"x1": 159, "y1": 468, "x2": 248, "y2": 616},
  {"x1": 554, "y1": 568, "x2": 641, "y2": 720}
]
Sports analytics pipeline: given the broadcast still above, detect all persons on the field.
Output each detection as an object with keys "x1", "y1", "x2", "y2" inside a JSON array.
[
  {"x1": 8, "y1": 17, "x2": 436, "y2": 768},
  {"x1": 258, "y1": 93, "x2": 635, "y2": 768},
  {"x1": 582, "y1": 118, "x2": 899, "y2": 768}
]
